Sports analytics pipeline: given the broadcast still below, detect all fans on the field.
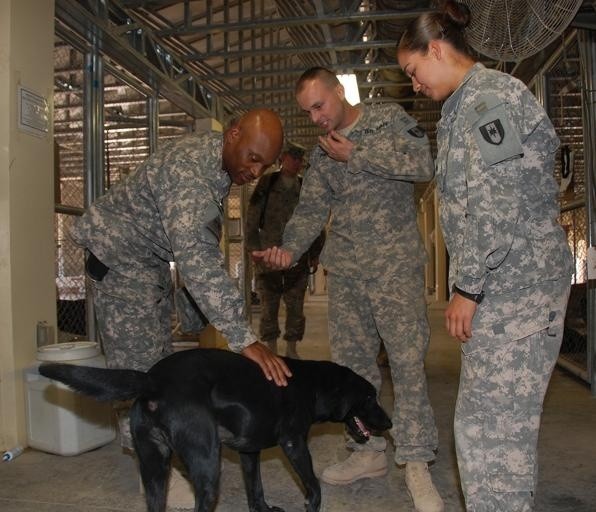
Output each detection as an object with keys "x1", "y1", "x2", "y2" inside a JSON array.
[{"x1": 452, "y1": 0, "x2": 584, "y2": 61}]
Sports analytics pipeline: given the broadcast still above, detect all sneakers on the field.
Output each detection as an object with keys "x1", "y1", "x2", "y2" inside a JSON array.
[
  {"x1": 405, "y1": 460, "x2": 445, "y2": 511},
  {"x1": 322, "y1": 449, "x2": 387, "y2": 486},
  {"x1": 140, "y1": 467, "x2": 196, "y2": 509}
]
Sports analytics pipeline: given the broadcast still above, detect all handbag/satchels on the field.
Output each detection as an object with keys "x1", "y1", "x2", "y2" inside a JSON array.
[{"x1": 268, "y1": 267, "x2": 299, "y2": 289}]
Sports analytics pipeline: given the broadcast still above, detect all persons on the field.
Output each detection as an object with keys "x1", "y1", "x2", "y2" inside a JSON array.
[
  {"x1": 243, "y1": 144, "x2": 325, "y2": 361},
  {"x1": 74, "y1": 109, "x2": 293, "y2": 509},
  {"x1": 395, "y1": 3, "x2": 575, "y2": 511},
  {"x1": 252, "y1": 67, "x2": 444, "y2": 512}
]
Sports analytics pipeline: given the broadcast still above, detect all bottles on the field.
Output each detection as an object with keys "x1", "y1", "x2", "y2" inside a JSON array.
[
  {"x1": 36, "y1": 320, "x2": 48, "y2": 347},
  {"x1": 2, "y1": 446, "x2": 24, "y2": 461}
]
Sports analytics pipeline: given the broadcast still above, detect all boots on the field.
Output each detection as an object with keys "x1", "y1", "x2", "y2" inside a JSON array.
[
  {"x1": 286, "y1": 341, "x2": 301, "y2": 359},
  {"x1": 265, "y1": 339, "x2": 277, "y2": 354}
]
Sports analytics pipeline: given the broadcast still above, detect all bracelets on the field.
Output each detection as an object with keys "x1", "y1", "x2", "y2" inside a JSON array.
[{"x1": 453, "y1": 284, "x2": 486, "y2": 305}]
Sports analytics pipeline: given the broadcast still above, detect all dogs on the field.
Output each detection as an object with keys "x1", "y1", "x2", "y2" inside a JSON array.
[{"x1": 38, "y1": 346, "x2": 393, "y2": 512}]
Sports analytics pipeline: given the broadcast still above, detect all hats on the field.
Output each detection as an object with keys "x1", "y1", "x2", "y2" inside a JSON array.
[{"x1": 283, "y1": 139, "x2": 306, "y2": 157}]
